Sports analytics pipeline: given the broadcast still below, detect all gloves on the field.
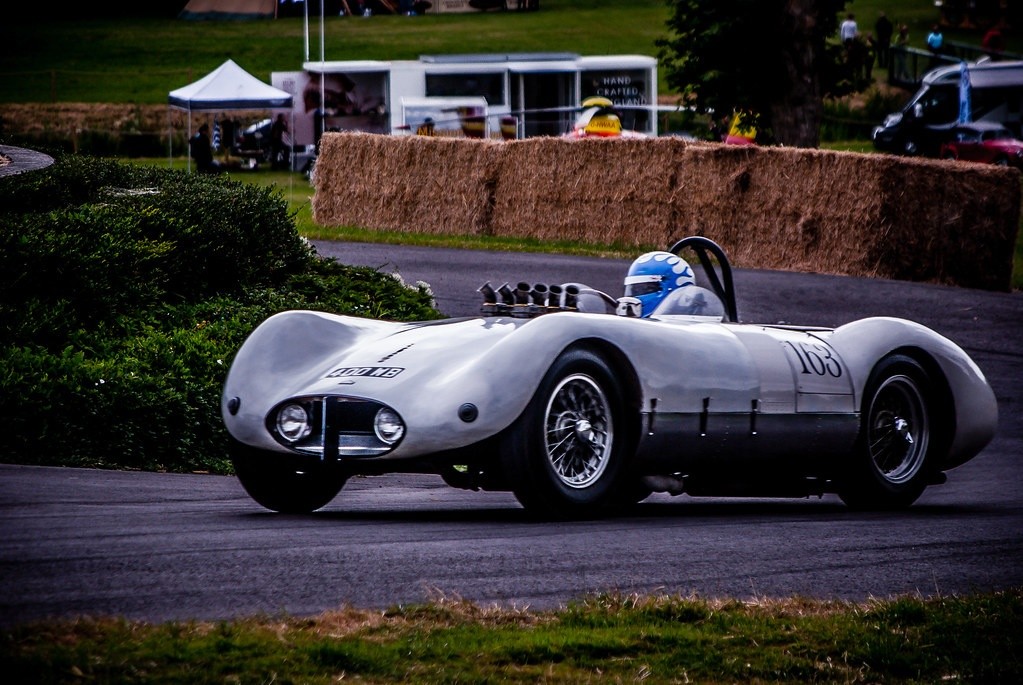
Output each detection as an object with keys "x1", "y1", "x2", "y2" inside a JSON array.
[{"x1": 615, "y1": 297, "x2": 642, "y2": 317}]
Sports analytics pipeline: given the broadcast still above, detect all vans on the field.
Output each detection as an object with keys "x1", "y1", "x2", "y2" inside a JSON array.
[{"x1": 872, "y1": 58, "x2": 1023, "y2": 158}]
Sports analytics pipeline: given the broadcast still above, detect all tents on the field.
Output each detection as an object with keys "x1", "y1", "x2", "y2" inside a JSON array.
[{"x1": 168, "y1": 58, "x2": 293, "y2": 172}]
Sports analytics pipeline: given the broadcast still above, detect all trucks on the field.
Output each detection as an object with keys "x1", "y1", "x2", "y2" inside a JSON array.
[{"x1": 271, "y1": 48, "x2": 658, "y2": 180}]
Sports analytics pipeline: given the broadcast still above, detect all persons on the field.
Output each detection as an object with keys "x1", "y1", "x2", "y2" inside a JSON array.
[
  {"x1": 922, "y1": 24, "x2": 943, "y2": 73},
  {"x1": 219, "y1": 112, "x2": 241, "y2": 161},
  {"x1": 314, "y1": 102, "x2": 328, "y2": 146},
  {"x1": 839, "y1": 14, "x2": 862, "y2": 47},
  {"x1": 864, "y1": 32, "x2": 875, "y2": 79},
  {"x1": 269, "y1": 114, "x2": 291, "y2": 171},
  {"x1": 893, "y1": 22, "x2": 911, "y2": 81},
  {"x1": 874, "y1": 10, "x2": 892, "y2": 67},
  {"x1": 189, "y1": 123, "x2": 213, "y2": 172},
  {"x1": 981, "y1": 21, "x2": 1006, "y2": 60},
  {"x1": 623, "y1": 250, "x2": 696, "y2": 316}
]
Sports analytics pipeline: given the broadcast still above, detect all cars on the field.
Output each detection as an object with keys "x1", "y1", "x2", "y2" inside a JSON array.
[
  {"x1": 941, "y1": 119, "x2": 1023, "y2": 174},
  {"x1": 223, "y1": 236, "x2": 996, "y2": 524}
]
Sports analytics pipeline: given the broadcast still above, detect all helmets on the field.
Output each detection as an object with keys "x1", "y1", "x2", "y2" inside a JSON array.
[{"x1": 624, "y1": 251, "x2": 696, "y2": 319}]
